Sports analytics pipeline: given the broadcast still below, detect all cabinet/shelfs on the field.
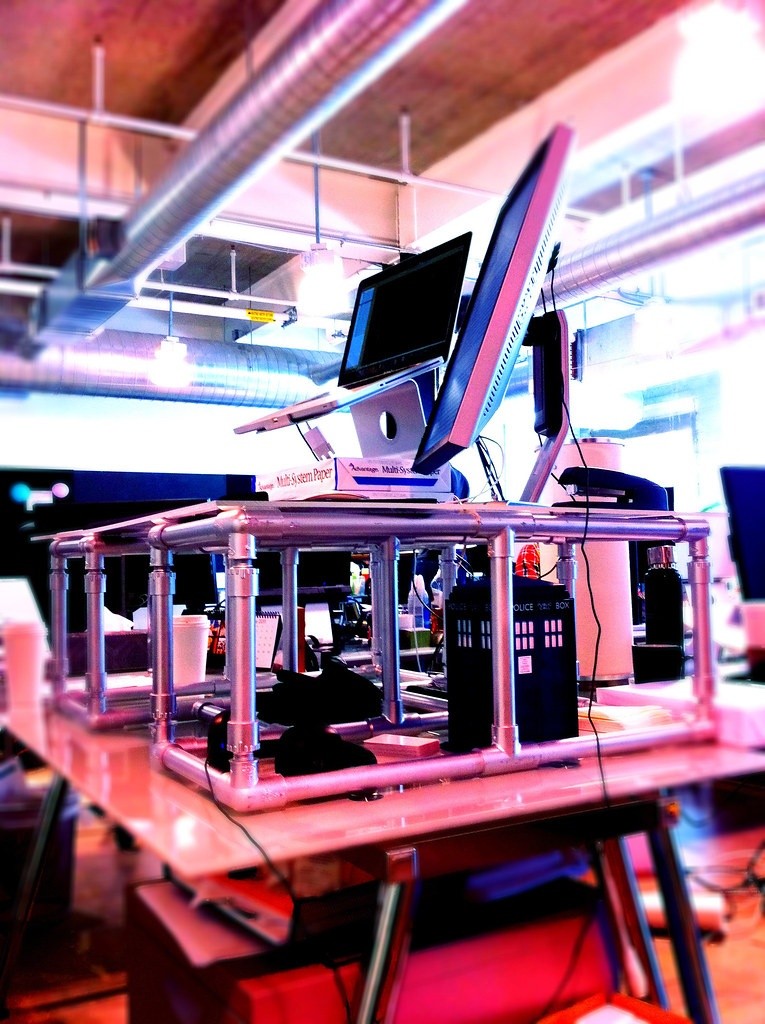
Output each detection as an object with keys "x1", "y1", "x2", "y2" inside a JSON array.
[{"x1": 49, "y1": 508, "x2": 720, "y2": 811}]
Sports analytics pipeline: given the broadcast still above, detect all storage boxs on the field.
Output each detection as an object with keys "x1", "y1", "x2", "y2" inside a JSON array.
[{"x1": 256, "y1": 457, "x2": 451, "y2": 492}]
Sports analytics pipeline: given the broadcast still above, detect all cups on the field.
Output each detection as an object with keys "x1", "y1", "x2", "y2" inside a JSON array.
[
  {"x1": 740, "y1": 604, "x2": 765, "y2": 670},
  {"x1": 173, "y1": 614, "x2": 210, "y2": 688},
  {"x1": 0, "y1": 622, "x2": 47, "y2": 708}
]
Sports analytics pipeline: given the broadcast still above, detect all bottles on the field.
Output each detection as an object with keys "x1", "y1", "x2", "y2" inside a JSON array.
[
  {"x1": 409, "y1": 574, "x2": 424, "y2": 628},
  {"x1": 417, "y1": 574, "x2": 430, "y2": 628},
  {"x1": 645, "y1": 546, "x2": 685, "y2": 676}
]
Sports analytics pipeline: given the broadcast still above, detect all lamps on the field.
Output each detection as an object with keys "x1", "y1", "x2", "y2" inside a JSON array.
[
  {"x1": 296, "y1": 130, "x2": 350, "y2": 324},
  {"x1": 151, "y1": 271, "x2": 191, "y2": 388}
]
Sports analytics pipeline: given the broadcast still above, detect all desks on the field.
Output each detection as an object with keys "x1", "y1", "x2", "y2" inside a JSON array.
[
  {"x1": 597, "y1": 678, "x2": 765, "y2": 750},
  {"x1": 0, "y1": 694, "x2": 765, "y2": 1023}
]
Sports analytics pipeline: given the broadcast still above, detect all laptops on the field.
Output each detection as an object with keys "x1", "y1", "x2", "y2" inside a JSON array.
[{"x1": 233, "y1": 230, "x2": 474, "y2": 435}]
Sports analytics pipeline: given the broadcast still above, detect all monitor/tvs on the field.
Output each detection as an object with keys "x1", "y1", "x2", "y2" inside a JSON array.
[{"x1": 412, "y1": 123, "x2": 577, "y2": 507}]
